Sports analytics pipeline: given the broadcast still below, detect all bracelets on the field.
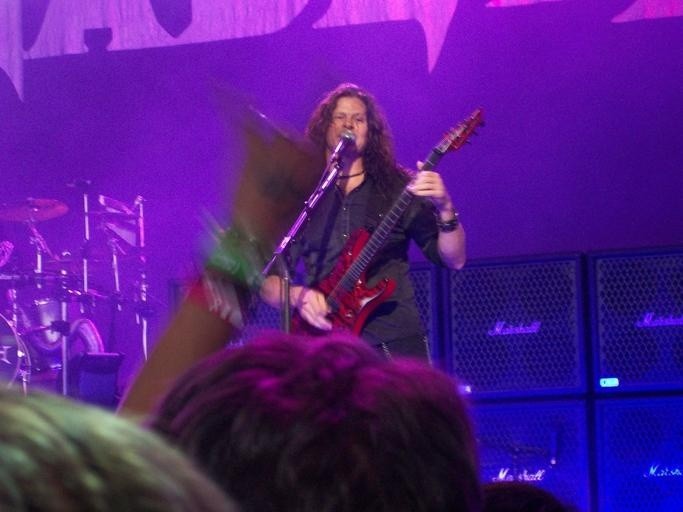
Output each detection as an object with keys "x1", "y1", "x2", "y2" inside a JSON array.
[{"x1": 434, "y1": 207, "x2": 457, "y2": 232}]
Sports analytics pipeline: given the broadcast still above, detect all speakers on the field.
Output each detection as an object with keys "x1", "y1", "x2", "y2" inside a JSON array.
[
  {"x1": 408, "y1": 260, "x2": 439, "y2": 373},
  {"x1": 595, "y1": 397, "x2": 683, "y2": 512},
  {"x1": 442, "y1": 251, "x2": 588, "y2": 401},
  {"x1": 467, "y1": 401, "x2": 596, "y2": 512},
  {"x1": 590, "y1": 246, "x2": 683, "y2": 395},
  {"x1": 168, "y1": 270, "x2": 283, "y2": 353}
]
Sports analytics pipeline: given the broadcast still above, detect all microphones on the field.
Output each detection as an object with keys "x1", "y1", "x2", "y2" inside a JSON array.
[{"x1": 330, "y1": 132, "x2": 355, "y2": 163}]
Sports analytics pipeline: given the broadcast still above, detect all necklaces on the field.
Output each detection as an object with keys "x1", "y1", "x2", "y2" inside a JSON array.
[{"x1": 336, "y1": 170, "x2": 366, "y2": 179}]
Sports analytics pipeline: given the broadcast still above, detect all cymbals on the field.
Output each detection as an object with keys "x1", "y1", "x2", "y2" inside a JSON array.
[{"x1": 0, "y1": 198, "x2": 68, "y2": 221}]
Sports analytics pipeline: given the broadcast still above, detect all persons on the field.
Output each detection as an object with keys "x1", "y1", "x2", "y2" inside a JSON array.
[
  {"x1": 0, "y1": 388, "x2": 237, "y2": 512},
  {"x1": 482, "y1": 481, "x2": 563, "y2": 512},
  {"x1": 256, "y1": 82, "x2": 467, "y2": 366},
  {"x1": 146, "y1": 334, "x2": 480, "y2": 511}
]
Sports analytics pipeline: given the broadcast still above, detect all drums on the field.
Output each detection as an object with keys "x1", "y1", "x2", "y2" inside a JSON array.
[
  {"x1": 23, "y1": 298, "x2": 62, "y2": 345},
  {"x1": 0, "y1": 314, "x2": 31, "y2": 394}
]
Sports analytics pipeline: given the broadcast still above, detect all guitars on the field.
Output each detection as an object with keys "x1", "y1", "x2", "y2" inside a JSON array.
[{"x1": 291, "y1": 105, "x2": 486, "y2": 339}]
